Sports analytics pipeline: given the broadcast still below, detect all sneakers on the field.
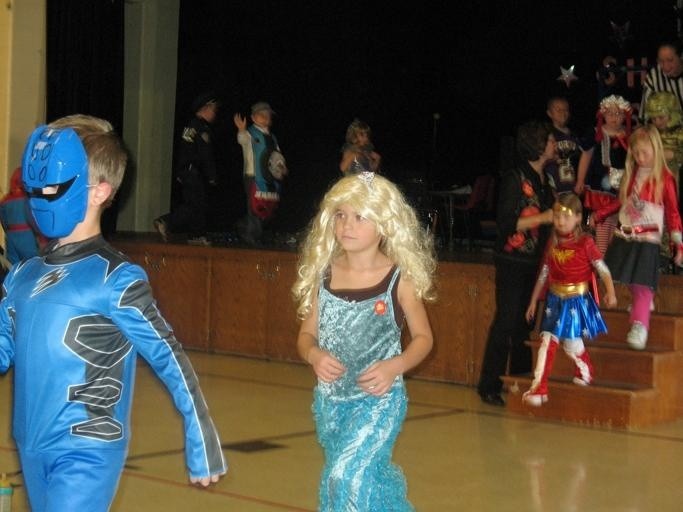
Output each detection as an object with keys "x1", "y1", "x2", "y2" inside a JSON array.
[
  {"x1": 153, "y1": 219, "x2": 169, "y2": 243},
  {"x1": 187, "y1": 233, "x2": 213, "y2": 246},
  {"x1": 626, "y1": 321, "x2": 649, "y2": 351}
]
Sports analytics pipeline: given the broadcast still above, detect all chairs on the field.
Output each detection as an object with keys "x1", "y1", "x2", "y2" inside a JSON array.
[{"x1": 428, "y1": 173, "x2": 493, "y2": 260}]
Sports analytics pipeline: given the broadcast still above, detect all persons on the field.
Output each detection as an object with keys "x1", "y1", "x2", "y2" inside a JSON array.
[
  {"x1": 291, "y1": 172, "x2": 436, "y2": 512},
  {"x1": 233, "y1": 102, "x2": 287, "y2": 250},
  {"x1": 0, "y1": 114, "x2": 228, "y2": 512},
  {"x1": 153, "y1": 91, "x2": 220, "y2": 246},
  {"x1": 0, "y1": 167, "x2": 49, "y2": 265},
  {"x1": 339, "y1": 121, "x2": 381, "y2": 176}
]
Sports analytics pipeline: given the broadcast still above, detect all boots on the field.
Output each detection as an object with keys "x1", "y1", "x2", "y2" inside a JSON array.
[
  {"x1": 519, "y1": 330, "x2": 561, "y2": 407},
  {"x1": 562, "y1": 344, "x2": 595, "y2": 386}
]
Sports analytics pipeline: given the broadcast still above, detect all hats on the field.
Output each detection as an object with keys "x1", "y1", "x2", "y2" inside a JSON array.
[{"x1": 251, "y1": 102, "x2": 274, "y2": 113}]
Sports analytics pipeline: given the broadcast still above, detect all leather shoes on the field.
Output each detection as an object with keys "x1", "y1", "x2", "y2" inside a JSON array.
[{"x1": 478, "y1": 389, "x2": 505, "y2": 407}]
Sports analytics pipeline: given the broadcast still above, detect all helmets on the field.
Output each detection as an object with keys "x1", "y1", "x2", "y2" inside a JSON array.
[{"x1": 645, "y1": 91, "x2": 683, "y2": 129}]
